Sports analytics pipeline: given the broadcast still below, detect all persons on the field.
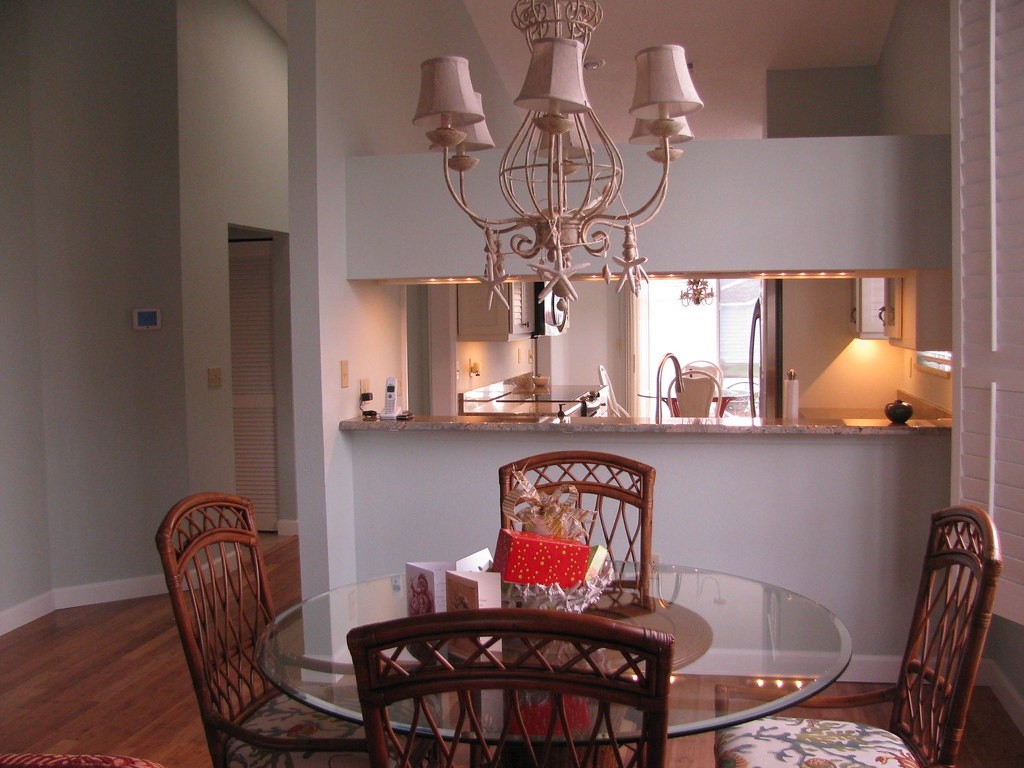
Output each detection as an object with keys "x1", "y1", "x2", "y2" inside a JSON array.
[{"x1": 410, "y1": 573, "x2": 432, "y2": 612}]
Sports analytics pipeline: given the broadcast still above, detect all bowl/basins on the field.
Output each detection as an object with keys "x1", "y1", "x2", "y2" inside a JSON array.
[
  {"x1": 512, "y1": 377, "x2": 528, "y2": 387},
  {"x1": 533, "y1": 377, "x2": 550, "y2": 386}
]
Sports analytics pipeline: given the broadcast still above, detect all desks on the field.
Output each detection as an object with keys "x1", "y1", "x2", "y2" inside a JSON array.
[
  {"x1": 252, "y1": 560, "x2": 853, "y2": 768},
  {"x1": 637, "y1": 387, "x2": 758, "y2": 418}
]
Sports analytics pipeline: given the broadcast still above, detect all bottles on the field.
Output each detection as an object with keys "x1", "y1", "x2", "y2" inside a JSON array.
[{"x1": 884, "y1": 399, "x2": 913, "y2": 425}]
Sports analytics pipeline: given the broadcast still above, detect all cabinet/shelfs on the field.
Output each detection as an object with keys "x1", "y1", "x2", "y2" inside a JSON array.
[
  {"x1": 878, "y1": 275, "x2": 952, "y2": 352},
  {"x1": 456, "y1": 282, "x2": 535, "y2": 343},
  {"x1": 569, "y1": 392, "x2": 607, "y2": 418},
  {"x1": 850, "y1": 277, "x2": 889, "y2": 340}
]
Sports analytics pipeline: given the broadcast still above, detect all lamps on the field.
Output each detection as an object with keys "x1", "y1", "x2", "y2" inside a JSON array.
[
  {"x1": 411, "y1": 0, "x2": 707, "y2": 311},
  {"x1": 679, "y1": 278, "x2": 715, "y2": 307}
]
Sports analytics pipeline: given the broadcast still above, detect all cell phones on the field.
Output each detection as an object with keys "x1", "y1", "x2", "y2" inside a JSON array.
[{"x1": 397, "y1": 413, "x2": 415, "y2": 419}]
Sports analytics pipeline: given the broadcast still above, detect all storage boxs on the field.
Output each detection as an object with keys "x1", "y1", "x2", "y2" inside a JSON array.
[{"x1": 491, "y1": 530, "x2": 590, "y2": 590}]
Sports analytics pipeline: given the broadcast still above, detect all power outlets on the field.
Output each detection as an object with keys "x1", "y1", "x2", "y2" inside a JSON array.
[
  {"x1": 207, "y1": 365, "x2": 224, "y2": 387},
  {"x1": 651, "y1": 554, "x2": 661, "y2": 578},
  {"x1": 528, "y1": 349, "x2": 534, "y2": 363},
  {"x1": 360, "y1": 378, "x2": 371, "y2": 405},
  {"x1": 518, "y1": 348, "x2": 522, "y2": 363},
  {"x1": 469, "y1": 359, "x2": 475, "y2": 377}
]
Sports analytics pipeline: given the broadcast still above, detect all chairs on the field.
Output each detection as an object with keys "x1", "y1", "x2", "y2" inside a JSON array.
[
  {"x1": 346, "y1": 606, "x2": 675, "y2": 768},
  {"x1": 681, "y1": 359, "x2": 724, "y2": 390},
  {"x1": 713, "y1": 505, "x2": 1002, "y2": 768},
  {"x1": 153, "y1": 492, "x2": 444, "y2": 768},
  {"x1": 497, "y1": 450, "x2": 656, "y2": 565},
  {"x1": 667, "y1": 370, "x2": 722, "y2": 417},
  {"x1": 599, "y1": 365, "x2": 631, "y2": 417}
]
